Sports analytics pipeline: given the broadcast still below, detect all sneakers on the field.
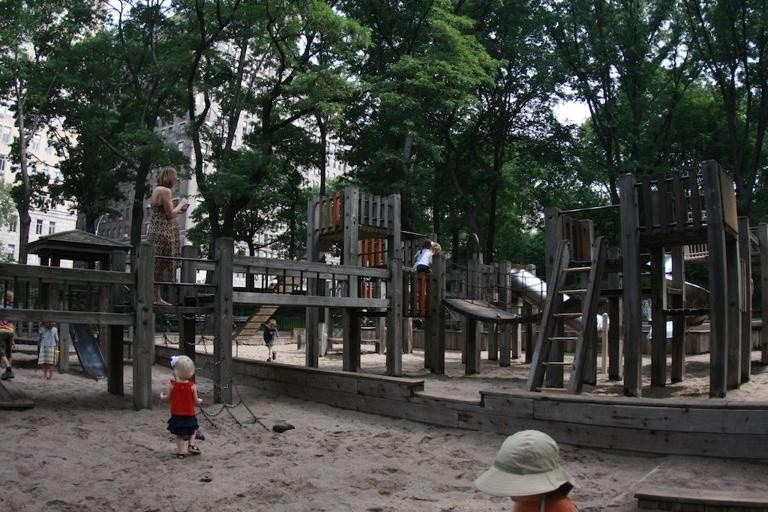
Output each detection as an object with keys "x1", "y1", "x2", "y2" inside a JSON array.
[
  {"x1": 267, "y1": 351, "x2": 276, "y2": 362},
  {"x1": 176, "y1": 445, "x2": 200, "y2": 459},
  {"x1": 2, "y1": 372, "x2": 15, "y2": 379}
]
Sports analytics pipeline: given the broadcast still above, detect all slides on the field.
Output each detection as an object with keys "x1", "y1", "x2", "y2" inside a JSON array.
[
  {"x1": 232, "y1": 236, "x2": 330, "y2": 341},
  {"x1": 69, "y1": 321, "x2": 108, "y2": 378},
  {"x1": 643, "y1": 252, "x2": 712, "y2": 330},
  {"x1": 511, "y1": 268, "x2": 611, "y2": 343}
]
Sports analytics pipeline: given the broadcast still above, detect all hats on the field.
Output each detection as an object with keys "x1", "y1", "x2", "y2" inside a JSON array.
[{"x1": 474, "y1": 429, "x2": 574, "y2": 496}]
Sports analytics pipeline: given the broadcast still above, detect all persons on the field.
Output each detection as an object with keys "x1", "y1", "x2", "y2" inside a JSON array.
[
  {"x1": 37, "y1": 318, "x2": 59, "y2": 379},
  {"x1": 0, "y1": 290, "x2": 18, "y2": 380},
  {"x1": 260, "y1": 318, "x2": 280, "y2": 362},
  {"x1": 147, "y1": 165, "x2": 189, "y2": 307},
  {"x1": 159, "y1": 355, "x2": 204, "y2": 458},
  {"x1": 411, "y1": 239, "x2": 443, "y2": 274},
  {"x1": 474, "y1": 429, "x2": 579, "y2": 512}
]
